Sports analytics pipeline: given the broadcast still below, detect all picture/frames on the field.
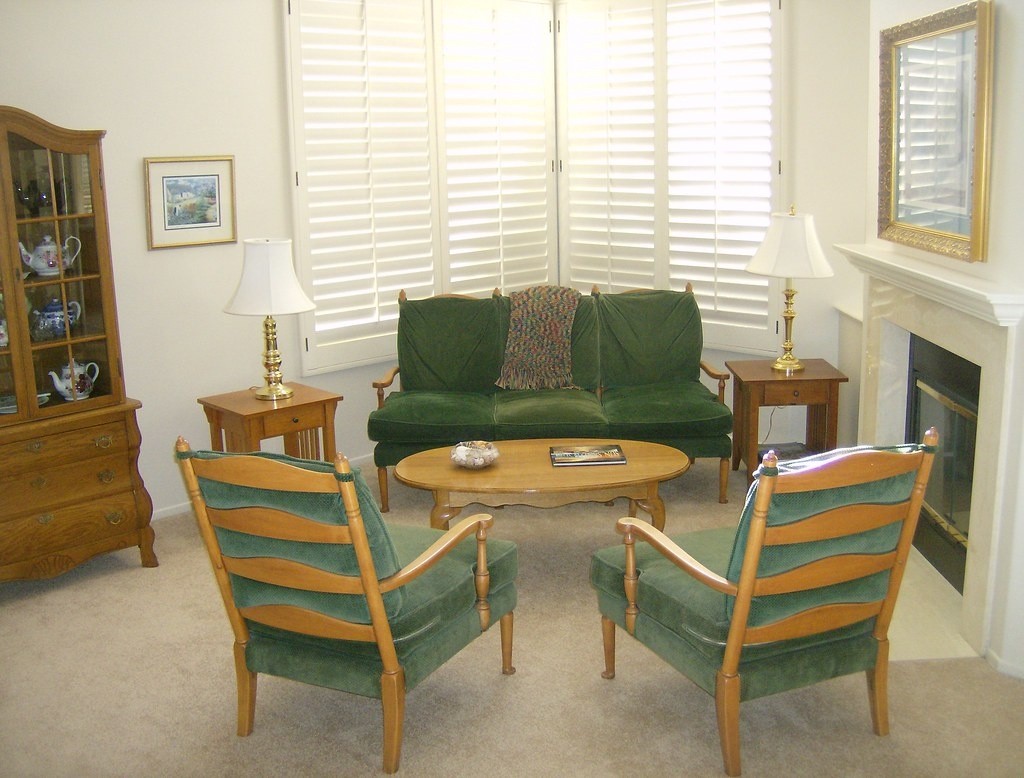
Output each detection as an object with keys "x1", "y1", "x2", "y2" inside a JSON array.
[
  {"x1": 143, "y1": 156, "x2": 238, "y2": 250},
  {"x1": 878, "y1": 0, "x2": 996, "y2": 262}
]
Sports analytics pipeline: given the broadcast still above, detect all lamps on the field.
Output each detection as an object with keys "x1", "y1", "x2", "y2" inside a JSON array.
[
  {"x1": 746, "y1": 204, "x2": 834, "y2": 371},
  {"x1": 223, "y1": 240, "x2": 317, "y2": 401}
]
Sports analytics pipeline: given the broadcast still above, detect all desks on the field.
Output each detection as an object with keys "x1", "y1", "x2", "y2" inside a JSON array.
[
  {"x1": 198, "y1": 383, "x2": 343, "y2": 462},
  {"x1": 726, "y1": 360, "x2": 849, "y2": 487}
]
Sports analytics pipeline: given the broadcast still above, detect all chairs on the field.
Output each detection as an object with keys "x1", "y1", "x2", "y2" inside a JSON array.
[
  {"x1": 174, "y1": 436, "x2": 521, "y2": 771},
  {"x1": 588, "y1": 426, "x2": 940, "y2": 777}
]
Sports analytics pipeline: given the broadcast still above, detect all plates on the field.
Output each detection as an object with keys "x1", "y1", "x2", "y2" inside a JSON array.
[{"x1": 0, "y1": 394, "x2": 50, "y2": 414}]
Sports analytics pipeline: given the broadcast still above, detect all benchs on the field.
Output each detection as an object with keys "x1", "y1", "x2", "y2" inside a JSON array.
[{"x1": 366, "y1": 282, "x2": 732, "y2": 513}]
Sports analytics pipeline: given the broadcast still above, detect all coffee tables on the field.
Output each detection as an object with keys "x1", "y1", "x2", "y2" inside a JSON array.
[{"x1": 394, "y1": 438, "x2": 690, "y2": 532}]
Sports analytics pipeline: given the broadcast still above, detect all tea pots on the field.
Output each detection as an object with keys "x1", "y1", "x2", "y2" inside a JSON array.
[
  {"x1": 18, "y1": 236, "x2": 81, "y2": 276},
  {"x1": 34, "y1": 299, "x2": 81, "y2": 335},
  {"x1": 49, "y1": 358, "x2": 99, "y2": 400},
  {"x1": 13, "y1": 175, "x2": 73, "y2": 215}
]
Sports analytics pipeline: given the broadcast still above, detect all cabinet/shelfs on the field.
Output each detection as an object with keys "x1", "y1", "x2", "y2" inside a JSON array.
[{"x1": 0, "y1": 106, "x2": 159, "y2": 582}]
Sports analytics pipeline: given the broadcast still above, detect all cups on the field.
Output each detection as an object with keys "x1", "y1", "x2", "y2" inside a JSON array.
[{"x1": 0, "y1": 319, "x2": 9, "y2": 347}]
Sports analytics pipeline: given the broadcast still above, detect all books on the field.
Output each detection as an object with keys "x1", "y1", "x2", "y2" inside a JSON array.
[{"x1": 549, "y1": 445, "x2": 627, "y2": 467}]
